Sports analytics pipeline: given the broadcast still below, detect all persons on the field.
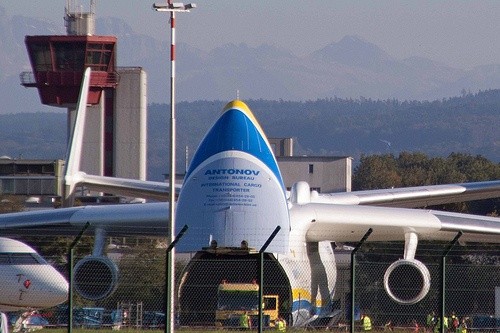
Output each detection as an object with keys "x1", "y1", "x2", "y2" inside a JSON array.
[
  {"x1": 111, "y1": 306, "x2": 123, "y2": 331},
  {"x1": 237, "y1": 310, "x2": 251, "y2": 332},
  {"x1": 359, "y1": 311, "x2": 473, "y2": 333},
  {"x1": 274, "y1": 315, "x2": 287, "y2": 333}
]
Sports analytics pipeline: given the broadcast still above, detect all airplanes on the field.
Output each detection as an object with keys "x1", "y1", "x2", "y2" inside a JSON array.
[
  {"x1": 0, "y1": 98, "x2": 499, "y2": 333},
  {"x1": 1, "y1": 235, "x2": 72, "y2": 332}
]
